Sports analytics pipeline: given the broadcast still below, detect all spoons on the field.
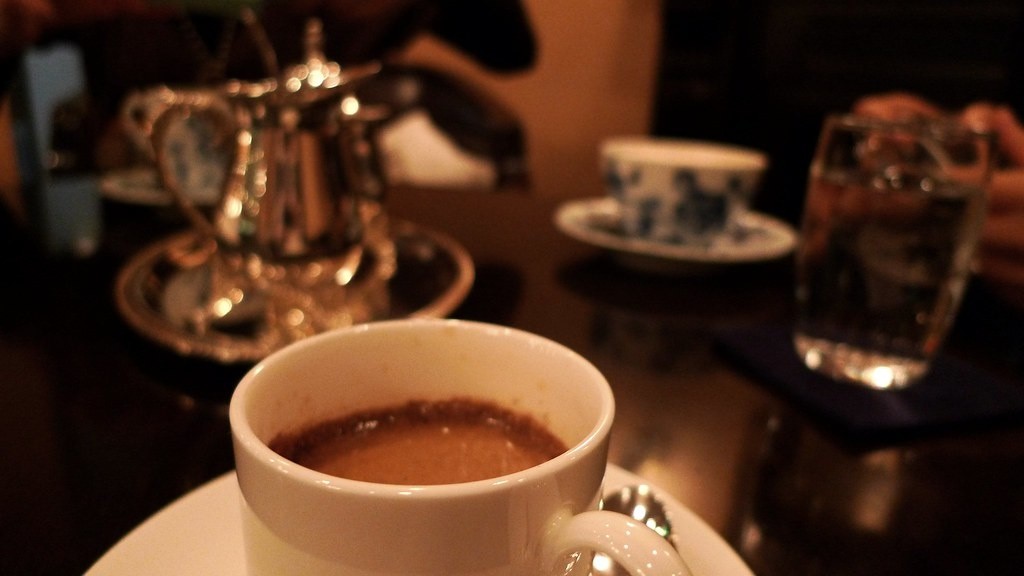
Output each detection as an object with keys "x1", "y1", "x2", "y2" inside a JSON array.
[{"x1": 599, "y1": 485, "x2": 671, "y2": 576}]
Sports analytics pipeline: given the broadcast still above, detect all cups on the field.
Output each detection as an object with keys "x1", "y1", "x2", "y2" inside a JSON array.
[
  {"x1": 601, "y1": 137, "x2": 767, "y2": 246},
  {"x1": 230, "y1": 318, "x2": 693, "y2": 576},
  {"x1": 795, "y1": 112, "x2": 993, "y2": 391}
]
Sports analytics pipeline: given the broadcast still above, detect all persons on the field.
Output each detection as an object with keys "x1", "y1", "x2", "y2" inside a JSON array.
[{"x1": 851, "y1": 88, "x2": 1024, "y2": 313}]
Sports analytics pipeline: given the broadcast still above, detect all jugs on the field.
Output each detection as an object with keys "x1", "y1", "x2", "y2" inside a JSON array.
[{"x1": 123, "y1": 18, "x2": 402, "y2": 318}]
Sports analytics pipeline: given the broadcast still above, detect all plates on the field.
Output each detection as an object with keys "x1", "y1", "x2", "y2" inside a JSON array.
[
  {"x1": 83, "y1": 461, "x2": 754, "y2": 576},
  {"x1": 114, "y1": 220, "x2": 476, "y2": 359},
  {"x1": 552, "y1": 196, "x2": 798, "y2": 261}
]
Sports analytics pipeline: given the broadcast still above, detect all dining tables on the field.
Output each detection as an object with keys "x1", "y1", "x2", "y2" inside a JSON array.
[{"x1": 1, "y1": 175, "x2": 1023, "y2": 575}]
